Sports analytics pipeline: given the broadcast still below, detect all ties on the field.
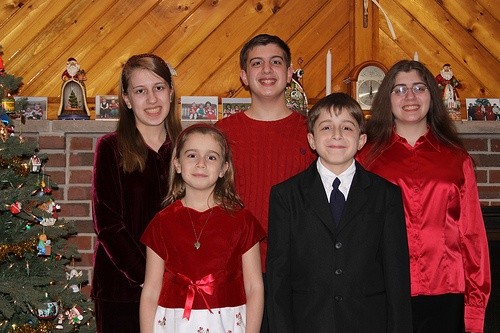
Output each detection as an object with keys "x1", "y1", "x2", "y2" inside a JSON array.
[{"x1": 331, "y1": 177, "x2": 345, "y2": 230}]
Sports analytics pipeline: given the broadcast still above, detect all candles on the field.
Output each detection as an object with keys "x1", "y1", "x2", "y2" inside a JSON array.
[
  {"x1": 326, "y1": 48, "x2": 332, "y2": 95},
  {"x1": 414, "y1": 51, "x2": 418, "y2": 60}
]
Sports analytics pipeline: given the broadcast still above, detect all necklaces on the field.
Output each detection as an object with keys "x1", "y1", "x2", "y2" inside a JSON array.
[{"x1": 185, "y1": 198, "x2": 214, "y2": 249}]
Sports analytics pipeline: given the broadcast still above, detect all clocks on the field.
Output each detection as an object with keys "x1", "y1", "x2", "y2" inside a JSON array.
[{"x1": 346, "y1": 59, "x2": 390, "y2": 118}]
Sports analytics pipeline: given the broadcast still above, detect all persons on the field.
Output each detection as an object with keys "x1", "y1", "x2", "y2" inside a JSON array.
[
  {"x1": 100, "y1": 97, "x2": 120, "y2": 119},
  {"x1": 38, "y1": 233, "x2": 52, "y2": 255},
  {"x1": 266, "y1": 92, "x2": 414, "y2": 333},
  {"x1": 224, "y1": 104, "x2": 251, "y2": 117},
  {"x1": 213, "y1": 35, "x2": 318, "y2": 285},
  {"x1": 137, "y1": 121, "x2": 264, "y2": 333},
  {"x1": 25, "y1": 103, "x2": 43, "y2": 120},
  {"x1": 92, "y1": 52, "x2": 185, "y2": 333},
  {"x1": 358, "y1": 59, "x2": 491, "y2": 333},
  {"x1": 189, "y1": 101, "x2": 216, "y2": 119},
  {"x1": 469, "y1": 102, "x2": 500, "y2": 120}
]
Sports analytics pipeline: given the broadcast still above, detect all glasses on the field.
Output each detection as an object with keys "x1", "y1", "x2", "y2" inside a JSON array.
[{"x1": 392, "y1": 85, "x2": 428, "y2": 95}]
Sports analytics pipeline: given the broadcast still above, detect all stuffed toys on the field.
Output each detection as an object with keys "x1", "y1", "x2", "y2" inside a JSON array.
[
  {"x1": 40, "y1": 201, "x2": 61, "y2": 226},
  {"x1": 62, "y1": 58, "x2": 85, "y2": 86},
  {"x1": 434, "y1": 64, "x2": 463, "y2": 109},
  {"x1": 68, "y1": 307, "x2": 83, "y2": 325}
]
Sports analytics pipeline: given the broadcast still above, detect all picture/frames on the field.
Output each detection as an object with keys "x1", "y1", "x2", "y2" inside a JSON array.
[
  {"x1": 94, "y1": 95, "x2": 120, "y2": 121},
  {"x1": 14, "y1": 96, "x2": 48, "y2": 119},
  {"x1": 222, "y1": 98, "x2": 251, "y2": 118},
  {"x1": 180, "y1": 96, "x2": 218, "y2": 121}
]
